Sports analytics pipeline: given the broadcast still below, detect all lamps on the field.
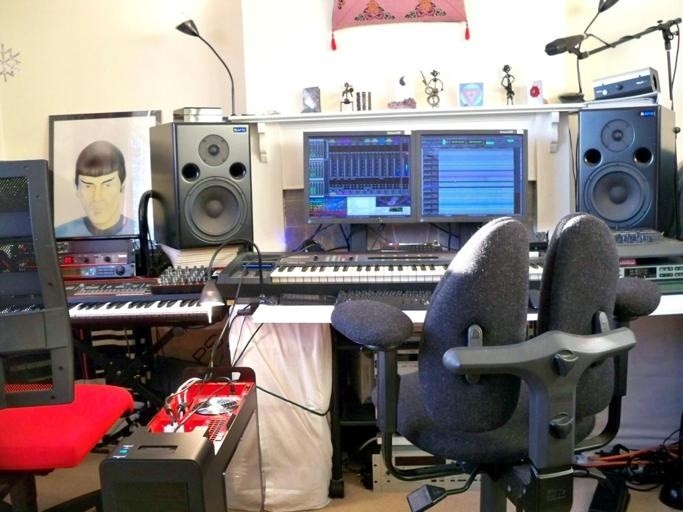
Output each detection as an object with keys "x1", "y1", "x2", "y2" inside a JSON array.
[
  {"x1": 174, "y1": 19, "x2": 237, "y2": 117},
  {"x1": 199, "y1": 238, "x2": 267, "y2": 309}
]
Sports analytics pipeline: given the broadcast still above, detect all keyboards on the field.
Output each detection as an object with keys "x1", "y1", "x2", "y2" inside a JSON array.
[{"x1": 335, "y1": 288, "x2": 436, "y2": 311}]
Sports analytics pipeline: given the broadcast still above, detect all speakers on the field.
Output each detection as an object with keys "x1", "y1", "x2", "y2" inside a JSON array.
[
  {"x1": 576, "y1": 105, "x2": 679, "y2": 240},
  {"x1": 149, "y1": 122, "x2": 254, "y2": 250}
]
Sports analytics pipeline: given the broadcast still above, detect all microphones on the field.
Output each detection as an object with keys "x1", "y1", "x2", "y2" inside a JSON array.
[{"x1": 544, "y1": 33, "x2": 588, "y2": 56}]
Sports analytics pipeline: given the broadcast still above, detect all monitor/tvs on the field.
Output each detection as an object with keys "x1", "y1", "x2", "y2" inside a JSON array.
[
  {"x1": 411, "y1": 128, "x2": 528, "y2": 251},
  {"x1": 302, "y1": 131, "x2": 416, "y2": 254}
]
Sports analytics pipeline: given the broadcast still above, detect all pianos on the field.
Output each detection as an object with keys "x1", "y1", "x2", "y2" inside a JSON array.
[
  {"x1": 216, "y1": 251, "x2": 546, "y2": 305},
  {"x1": 1, "y1": 277, "x2": 227, "y2": 330}
]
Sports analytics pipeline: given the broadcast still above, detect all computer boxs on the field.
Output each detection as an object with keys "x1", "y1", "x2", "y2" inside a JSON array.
[{"x1": 99, "y1": 367, "x2": 267, "y2": 512}]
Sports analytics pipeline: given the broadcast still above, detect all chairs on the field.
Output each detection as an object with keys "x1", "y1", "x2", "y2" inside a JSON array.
[
  {"x1": 1, "y1": 160, "x2": 136, "y2": 512},
  {"x1": 330, "y1": 212, "x2": 662, "y2": 512}
]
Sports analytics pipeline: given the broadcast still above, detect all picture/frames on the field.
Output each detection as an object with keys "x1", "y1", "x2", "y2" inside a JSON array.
[{"x1": 48, "y1": 109, "x2": 164, "y2": 243}]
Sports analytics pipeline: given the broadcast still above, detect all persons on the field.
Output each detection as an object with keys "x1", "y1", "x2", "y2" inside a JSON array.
[{"x1": 54, "y1": 140, "x2": 140, "y2": 237}]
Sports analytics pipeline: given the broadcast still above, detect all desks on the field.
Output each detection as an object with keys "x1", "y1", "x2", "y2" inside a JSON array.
[{"x1": 226, "y1": 293, "x2": 683, "y2": 511}]
